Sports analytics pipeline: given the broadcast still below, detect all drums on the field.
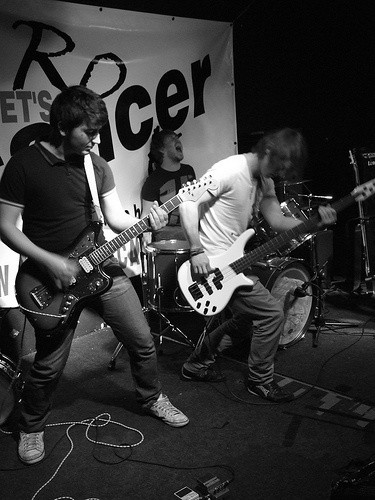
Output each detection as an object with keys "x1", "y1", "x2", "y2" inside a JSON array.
[
  {"x1": 232, "y1": 256, "x2": 315, "y2": 346},
  {"x1": 143, "y1": 239, "x2": 196, "y2": 313},
  {"x1": 252, "y1": 197, "x2": 318, "y2": 254}
]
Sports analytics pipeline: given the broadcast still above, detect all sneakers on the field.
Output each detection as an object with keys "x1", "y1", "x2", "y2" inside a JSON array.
[
  {"x1": 18, "y1": 431, "x2": 46, "y2": 465},
  {"x1": 248, "y1": 381, "x2": 293, "y2": 401},
  {"x1": 150, "y1": 393, "x2": 189, "y2": 427},
  {"x1": 181, "y1": 366, "x2": 224, "y2": 381}
]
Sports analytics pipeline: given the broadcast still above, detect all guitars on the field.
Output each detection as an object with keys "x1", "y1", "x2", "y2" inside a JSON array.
[
  {"x1": 15, "y1": 172, "x2": 220, "y2": 337},
  {"x1": 176, "y1": 180, "x2": 374, "y2": 317}
]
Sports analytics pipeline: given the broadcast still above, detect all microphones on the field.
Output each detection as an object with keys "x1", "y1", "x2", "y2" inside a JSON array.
[{"x1": 289, "y1": 286, "x2": 307, "y2": 298}]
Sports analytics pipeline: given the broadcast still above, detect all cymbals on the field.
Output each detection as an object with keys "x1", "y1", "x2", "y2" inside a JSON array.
[{"x1": 271, "y1": 180, "x2": 308, "y2": 186}]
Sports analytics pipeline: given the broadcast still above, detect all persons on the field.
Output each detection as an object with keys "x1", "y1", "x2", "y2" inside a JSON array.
[
  {"x1": 140, "y1": 130, "x2": 197, "y2": 244},
  {"x1": 179, "y1": 129, "x2": 338, "y2": 402},
  {"x1": 0, "y1": 85, "x2": 189, "y2": 466}
]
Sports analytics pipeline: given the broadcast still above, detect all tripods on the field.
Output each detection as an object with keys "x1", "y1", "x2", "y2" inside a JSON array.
[
  {"x1": 108, "y1": 238, "x2": 198, "y2": 369},
  {"x1": 301, "y1": 260, "x2": 358, "y2": 346}
]
